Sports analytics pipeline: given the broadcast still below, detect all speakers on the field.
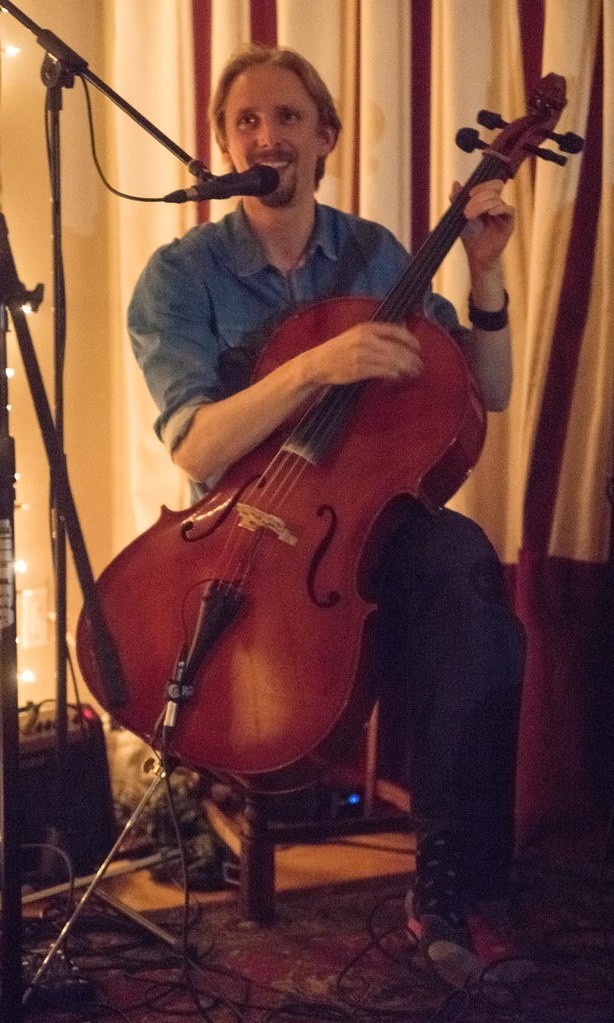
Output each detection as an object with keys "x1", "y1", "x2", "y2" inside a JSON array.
[{"x1": 17, "y1": 706, "x2": 119, "y2": 889}]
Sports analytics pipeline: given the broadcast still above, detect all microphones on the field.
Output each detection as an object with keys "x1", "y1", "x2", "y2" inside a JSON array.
[{"x1": 164, "y1": 163, "x2": 282, "y2": 204}]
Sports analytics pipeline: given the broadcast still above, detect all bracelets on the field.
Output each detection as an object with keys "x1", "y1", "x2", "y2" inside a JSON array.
[{"x1": 468, "y1": 290, "x2": 510, "y2": 331}]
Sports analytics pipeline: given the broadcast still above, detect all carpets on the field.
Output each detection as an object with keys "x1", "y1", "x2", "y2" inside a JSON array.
[{"x1": 20, "y1": 871, "x2": 614, "y2": 1023}]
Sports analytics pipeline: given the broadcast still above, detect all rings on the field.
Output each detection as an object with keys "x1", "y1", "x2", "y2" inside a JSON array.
[{"x1": 500, "y1": 200, "x2": 506, "y2": 207}]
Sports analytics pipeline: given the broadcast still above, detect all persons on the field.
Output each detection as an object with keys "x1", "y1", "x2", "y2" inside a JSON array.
[{"x1": 125, "y1": 49, "x2": 535, "y2": 1004}]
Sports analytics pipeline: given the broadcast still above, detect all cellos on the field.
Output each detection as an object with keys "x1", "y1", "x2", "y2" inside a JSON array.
[{"x1": 20, "y1": 73, "x2": 585, "y2": 1012}]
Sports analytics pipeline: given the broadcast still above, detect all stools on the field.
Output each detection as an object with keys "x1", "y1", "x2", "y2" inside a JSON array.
[{"x1": 194, "y1": 700, "x2": 412, "y2": 921}]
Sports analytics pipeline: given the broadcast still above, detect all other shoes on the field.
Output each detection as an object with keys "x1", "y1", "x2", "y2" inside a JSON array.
[
  {"x1": 405, "y1": 890, "x2": 480, "y2": 974},
  {"x1": 459, "y1": 897, "x2": 540, "y2": 988}
]
